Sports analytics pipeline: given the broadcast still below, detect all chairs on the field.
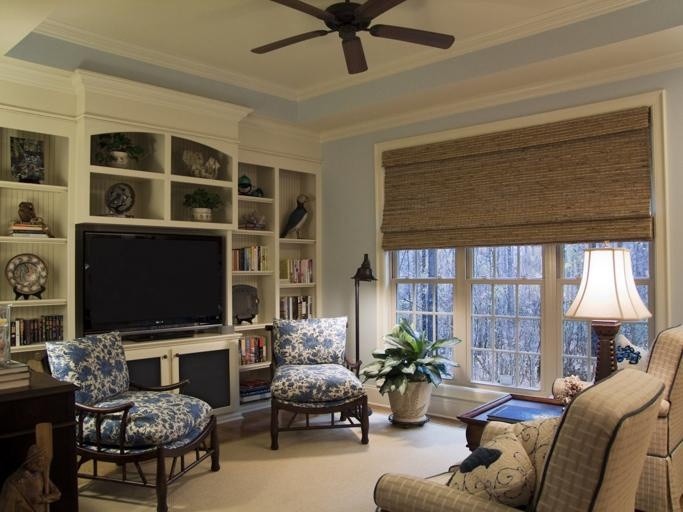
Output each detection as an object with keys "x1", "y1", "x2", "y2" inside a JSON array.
[
  {"x1": 260, "y1": 315, "x2": 372, "y2": 452},
  {"x1": 371, "y1": 364, "x2": 667, "y2": 510},
  {"x1": 619, "y1": 321, "x2": 683, "y2": 511},
  {"x1": 39, "y1": 328, "x2": 222, "y2": 511}
]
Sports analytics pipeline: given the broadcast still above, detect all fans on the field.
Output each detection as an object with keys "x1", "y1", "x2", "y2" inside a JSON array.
[{"x1": 242, "y1": 0, "x2": 455, "y2": 79}]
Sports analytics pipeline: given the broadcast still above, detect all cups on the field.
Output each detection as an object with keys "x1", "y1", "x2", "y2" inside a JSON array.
[{"x1": 0, "y1": 304, "x2": 11, "y2": 368}]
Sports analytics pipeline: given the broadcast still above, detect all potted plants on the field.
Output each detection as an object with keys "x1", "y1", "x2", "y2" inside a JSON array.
[{"x1": 353, "y1": 314, "x2": 464, "y2": 428}]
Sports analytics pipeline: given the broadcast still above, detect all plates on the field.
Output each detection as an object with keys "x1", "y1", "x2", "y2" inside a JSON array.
[
  {"x1": 4, "y1": 253, "x2": 48, "y2": 294},
  {"x1": 232, "y1": 284, "x2": 259, "y2": 318},
  {"x1": 105, "y1": 182, "x2": 136, "y2": 213}
]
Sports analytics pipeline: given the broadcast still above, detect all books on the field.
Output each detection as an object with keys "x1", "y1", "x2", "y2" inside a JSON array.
[
  {"x1": 9, "y1": 313, "x2": 63, "y2": 346},
  {"x1": 0, "y1": 371, "x2": 30, "y2": 381},
  {"x1": 8, "y1": 224, "x2": 50, "y2": 238},
  {"x1": 232, "y1": 245, "x2": 313, "y2": 403},
  {"x1": 0, "y1": 378, "x2": 31, "y2": 389},
  {"x1": 0, "y1": 356, "x2": 31, "y2": 374}
]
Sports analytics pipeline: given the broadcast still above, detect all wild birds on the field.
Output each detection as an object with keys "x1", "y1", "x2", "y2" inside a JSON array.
[{"x1": 280, "y1": 194, "x2": 312, "y2": 239}]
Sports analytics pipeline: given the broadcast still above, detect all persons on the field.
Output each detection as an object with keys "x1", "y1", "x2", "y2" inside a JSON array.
[{"x1": 0, "y1": 445, "x2": 61, "y2": 512}]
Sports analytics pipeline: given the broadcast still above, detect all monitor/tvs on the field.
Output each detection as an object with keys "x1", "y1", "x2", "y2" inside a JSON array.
[{"x1": 84, "y1": 231, "x2": 222, "y2": 341}]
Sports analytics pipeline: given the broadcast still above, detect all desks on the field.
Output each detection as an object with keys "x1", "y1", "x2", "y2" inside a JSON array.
[
  {"x1": 0, "y1": 361, "x2": 81, "y2": 511},
  {"x1": 452, "y1": 390, "x2": 570, "y2": 458}
]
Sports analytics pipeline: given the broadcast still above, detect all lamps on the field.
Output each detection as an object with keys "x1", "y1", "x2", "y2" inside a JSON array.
[
  {"x1": 559, "y1": 239, "x2": 654, "y2": 389},
  {"x1": 341, "y1": 250, "x2": 380, "y2": 420}
]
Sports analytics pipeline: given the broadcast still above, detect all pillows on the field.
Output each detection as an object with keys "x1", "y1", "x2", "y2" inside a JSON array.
[
  {"x1": 444, "y1": 428, "x2": 537, "y2": 509},
  {"x1": 497, "y1": 409, "x2": 563, "y2": 497},
  {"x1": 611, "y1": 332, "x2": 648, "y2": 373}
]
